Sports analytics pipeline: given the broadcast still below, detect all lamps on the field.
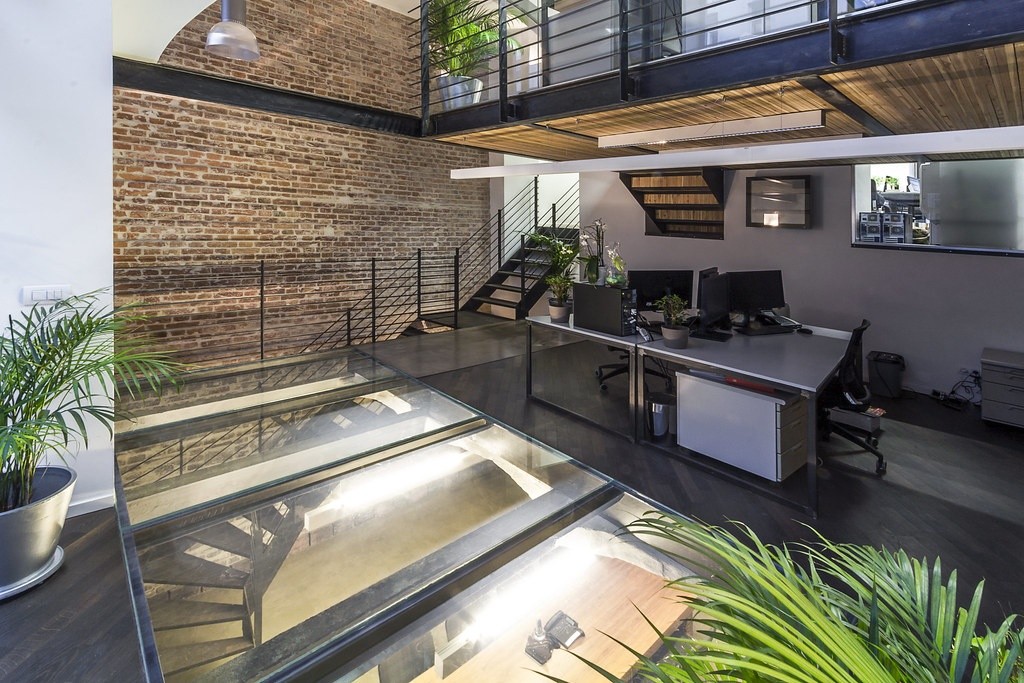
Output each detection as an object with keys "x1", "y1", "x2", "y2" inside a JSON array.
[{"x1": 204, "y1": 1, "x2": 260, "y2": 60}]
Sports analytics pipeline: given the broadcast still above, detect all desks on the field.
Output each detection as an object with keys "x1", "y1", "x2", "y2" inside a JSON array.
[
  {"x1": 524, "y1": 315, "x2": 852, "y2": 521},
  {"x1": 567, "y1": 558, "x2": 698, "y2": 683}
]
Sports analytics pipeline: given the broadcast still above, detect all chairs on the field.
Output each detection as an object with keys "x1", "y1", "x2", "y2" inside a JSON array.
[{"x1": 815, "y1": 319, "x2": 888, "y2": 477}]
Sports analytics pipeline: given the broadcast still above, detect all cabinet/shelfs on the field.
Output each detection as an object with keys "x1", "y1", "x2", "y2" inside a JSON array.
[{"x1": 979, "y1": 348, "x2": 1024, "y2": 430}]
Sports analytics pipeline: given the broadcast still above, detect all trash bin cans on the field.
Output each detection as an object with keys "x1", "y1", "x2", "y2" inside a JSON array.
[{"x1": 864, "y1": 350, "x2": 902, "y2": 398}]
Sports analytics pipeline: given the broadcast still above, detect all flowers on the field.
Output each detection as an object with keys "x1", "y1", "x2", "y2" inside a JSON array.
[{"x1": 578, "y1": 217, "x2": 628, "y2": 278}]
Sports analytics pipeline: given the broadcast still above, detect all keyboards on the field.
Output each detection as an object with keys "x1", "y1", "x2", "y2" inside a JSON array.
[{"x1": 732, "y1": 326, "x2": 795, "y2": 336}]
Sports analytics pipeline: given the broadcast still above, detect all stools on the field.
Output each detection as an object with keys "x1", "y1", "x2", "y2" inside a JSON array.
[{"x1": 593, "y1": 346, "x2": 672, "y2": 391}]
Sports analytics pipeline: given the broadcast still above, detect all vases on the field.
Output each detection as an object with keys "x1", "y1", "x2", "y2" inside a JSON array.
[{"x1": 596, "y1": 267, "x2": 606, "y2": 286}]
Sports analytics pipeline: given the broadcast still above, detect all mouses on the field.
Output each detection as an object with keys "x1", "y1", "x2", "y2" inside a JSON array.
[{"x1": 797, "y1": 328, "x2": 813, "y2": 335}]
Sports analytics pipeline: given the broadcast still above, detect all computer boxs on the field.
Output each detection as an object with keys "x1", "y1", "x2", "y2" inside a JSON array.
[{"x1": 573, "y1": 280, "x2": 639, "y2": 338}]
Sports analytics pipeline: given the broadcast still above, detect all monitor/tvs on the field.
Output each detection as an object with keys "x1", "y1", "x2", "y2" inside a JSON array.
[
  {"x1": 746, "y1": 176, "x2": 811, "y2": 229},
  {"x1": 629, "y1": 266, "x2": 785, "y2": 343}
]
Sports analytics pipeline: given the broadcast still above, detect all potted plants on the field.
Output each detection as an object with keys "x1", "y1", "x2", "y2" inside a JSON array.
[
  {"x1": 653, "y1": 293, "x2": 691, "y2": 350},
  {"x1": 428, "y1": 0, "x2": 528, "y2": 112},
  {"x1": 0, "y1": 280, "x2": 206, "y2": 601},
  {"x1": 514, "y1": 226, "x2": 579, "y2": 323}
]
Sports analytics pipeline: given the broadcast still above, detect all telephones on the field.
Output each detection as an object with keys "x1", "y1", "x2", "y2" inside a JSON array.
[{"x1": 756, "y1": 310, "x2": 802, "y2": 329}]
